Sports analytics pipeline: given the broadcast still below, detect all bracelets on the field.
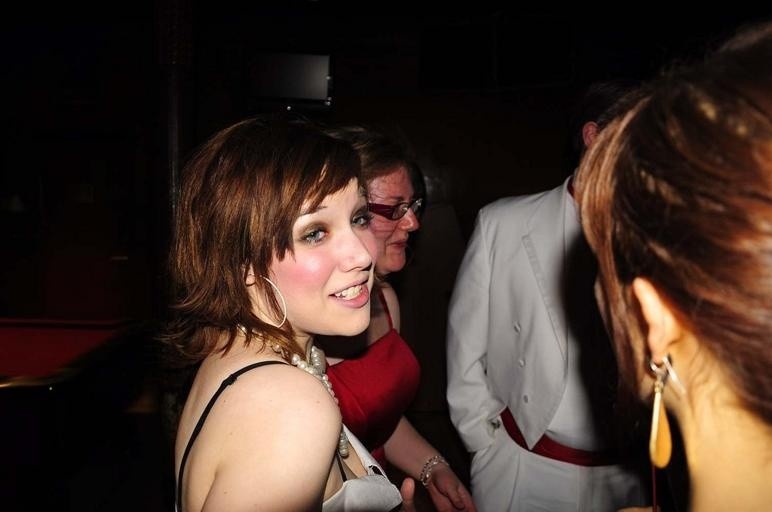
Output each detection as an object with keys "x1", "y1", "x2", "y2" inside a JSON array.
[{"x1": 419, "y1": 455, "x2": 449, "y2": 486}]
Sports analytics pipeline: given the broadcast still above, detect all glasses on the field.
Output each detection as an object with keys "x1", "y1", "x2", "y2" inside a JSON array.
[{"x1": 368, "y1": 197, "x2": 422, "y2": 221}]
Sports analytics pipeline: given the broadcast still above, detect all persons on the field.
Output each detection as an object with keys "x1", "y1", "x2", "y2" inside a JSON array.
[
  {"x1": 575, "y1": 23, "x2": 771, "y2": 511},
  {"x1": 168, "y1": 116, "x2": 414, "y2": 512},
  {"x1": 315, "y1": 114, "x2": 476, "y2": 511},
  {"x1": 446, "y1": 63, "x2": 674, "y2": 512}
]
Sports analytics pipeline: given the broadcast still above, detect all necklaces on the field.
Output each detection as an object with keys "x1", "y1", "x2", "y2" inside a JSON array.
[{"x1": 240, "y1": 322, "x2": 348, "y2": 458}]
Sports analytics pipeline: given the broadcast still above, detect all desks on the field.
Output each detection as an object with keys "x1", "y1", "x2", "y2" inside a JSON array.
[{"x1": 0, "y1": 317, "x2": 152, "y2": 476}]
